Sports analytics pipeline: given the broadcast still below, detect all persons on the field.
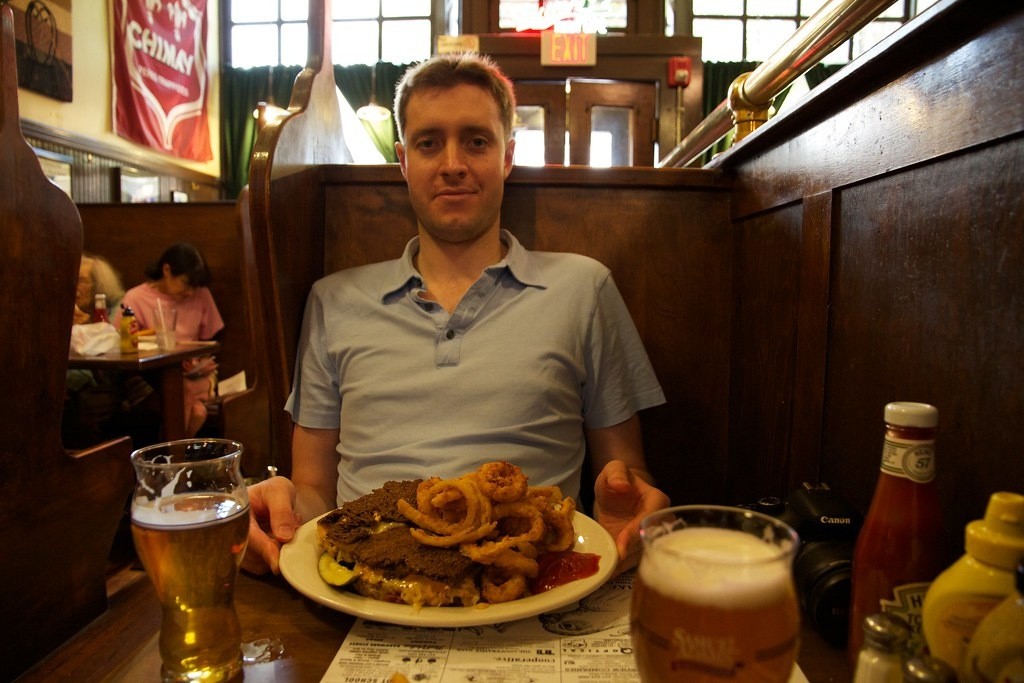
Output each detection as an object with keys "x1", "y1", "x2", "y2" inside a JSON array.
[
  {"x1": 242, "y1": 57, "x2": 675, "y2": 582},
  {"x1": 59, "y1": 245, "x2": 225, "y2": 439}
]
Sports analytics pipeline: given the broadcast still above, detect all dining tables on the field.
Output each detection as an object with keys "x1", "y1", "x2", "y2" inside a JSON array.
[
  {"x1": 66, "y1": 328, "x2": 225, "y2": 499},
  {"x1": 11, "y1": 569, "x2": 856, "y2": 683}
]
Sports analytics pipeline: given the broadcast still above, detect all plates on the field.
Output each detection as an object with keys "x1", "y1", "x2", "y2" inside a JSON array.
[{"x1": 277, "y1": 503, "x2": 616, "y2": 626}]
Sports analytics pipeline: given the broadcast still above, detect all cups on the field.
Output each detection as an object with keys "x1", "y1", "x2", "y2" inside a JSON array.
[
  {"x1": 131, "y1": 438, "x2": 249, "y2": 683},
  {"x1": 153, "y1": 307, "x2": 177, "y2": 348},
  {"x1": 629, "y1": 505, "x2": 799, "y2": 683}
]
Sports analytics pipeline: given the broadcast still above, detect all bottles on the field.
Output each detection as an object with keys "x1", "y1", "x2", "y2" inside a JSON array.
[
  {"x1": 856, "y1": 611, "x2": 955, "y2": 683},
  {"x1": 852, "y1": 402, "x2": 939, "y2": 668},
  {"x1": 119, "y1": 303, "x2": 138, "y2": 354},
  {"x1": 923, "y1": 492, "x2": 1024, "y2": 683},
  {"x1": 91, "y1": 294, "x2": 110, "y2": 324}
]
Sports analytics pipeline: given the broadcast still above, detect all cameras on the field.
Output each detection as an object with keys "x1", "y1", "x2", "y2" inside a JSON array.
[{"x1": 738, "y1": 485, "x2": 872, "y2": 651}]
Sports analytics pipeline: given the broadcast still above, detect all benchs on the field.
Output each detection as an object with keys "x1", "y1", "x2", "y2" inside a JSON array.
[
  {"x1": 59, "y1": 433, "x2": 134, "y2": 636},
  {"x1": 78, "y1": 197, "x2": 273, "y2": 485}
]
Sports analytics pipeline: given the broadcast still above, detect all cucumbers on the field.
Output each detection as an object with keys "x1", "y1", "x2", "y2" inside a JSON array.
[{"x1": 317, "y1": 550, "x2": 360, "y2": 587}]
